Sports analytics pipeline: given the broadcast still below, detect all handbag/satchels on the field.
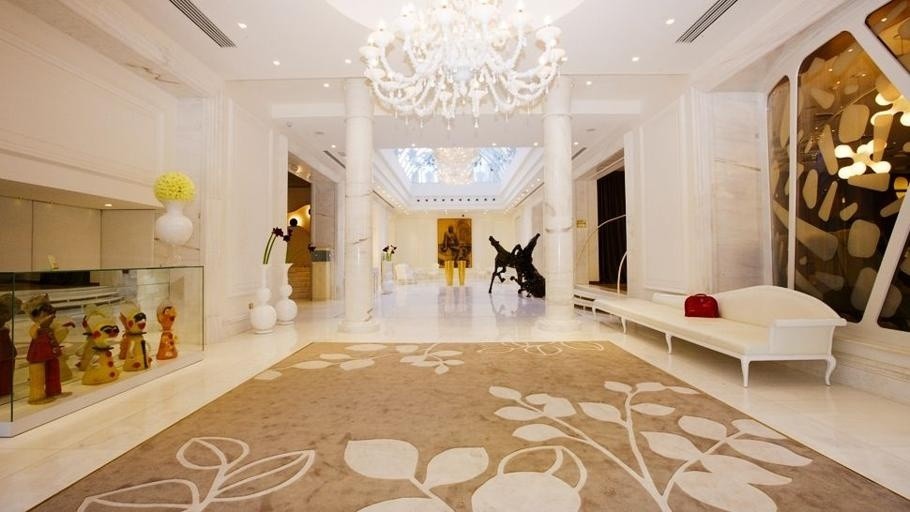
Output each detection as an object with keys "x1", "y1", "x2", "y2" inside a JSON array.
[{"x1": 685, "y1": 294, "x2": 719, "y2": 317}]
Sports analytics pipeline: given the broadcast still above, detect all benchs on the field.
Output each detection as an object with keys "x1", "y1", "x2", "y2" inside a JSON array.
[{"x1": 591, "y1": 284, "x2": 848, "y2": 389}]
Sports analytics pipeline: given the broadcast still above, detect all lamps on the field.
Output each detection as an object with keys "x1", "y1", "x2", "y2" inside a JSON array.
[{"x1": 357, "y1": 0, "x2": 566, "y2": 135}]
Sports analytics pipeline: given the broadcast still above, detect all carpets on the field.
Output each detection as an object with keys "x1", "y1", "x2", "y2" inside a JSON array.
[{"x1": 21, "y1": 340, "x2": 907, "y2": 510}]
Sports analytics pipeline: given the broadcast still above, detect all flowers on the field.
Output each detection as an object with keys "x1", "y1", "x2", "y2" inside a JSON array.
[
  {"x1": 151, "y1": 169, "x2": 196, "y2": 205},
  {"x1": 261, "y1": 227, "x2": 290, "y2": 265}
]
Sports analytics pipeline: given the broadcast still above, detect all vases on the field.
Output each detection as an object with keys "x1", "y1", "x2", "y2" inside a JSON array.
[
  {"x1": 155, "y1": 201, "x2": 192, "y2": 244},
  {"x1": 249, "y1": 262, "x2": 298, "y2": 335}
]
